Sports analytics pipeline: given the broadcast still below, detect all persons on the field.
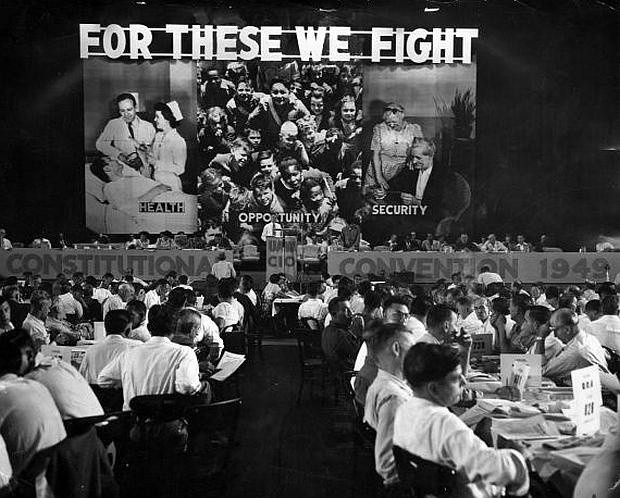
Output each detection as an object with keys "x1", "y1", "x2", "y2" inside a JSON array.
[
  {"x1": 363, "y1": 136, "x2": 457, "y2": 233},
  {"x1": 90, "y1": 150, "x2": 172, "y2": 218},
  {"x1": 95, "y1": 92, "x2": 156, "y2": 179},
  {"x1": 196, "y1": 58, "x2": 362, "y2": 246},
  {"x1": 1, "y1": 214, "x2": 619, "y2": 497},
  {"x1": 363, "y1": 102, "x2": 424, "y2": 195},
  {"x1": 135, "y1": 101, "x2": 188, "y2": 194}
]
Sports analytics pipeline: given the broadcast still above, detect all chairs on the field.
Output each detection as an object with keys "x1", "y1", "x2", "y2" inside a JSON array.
[
  {"x1": 390, "y1": 443, "x2": 459, "y2": 498},
  {"x1": 2, "y1": 393, "x2": 243, "y2": 495},
  {"x1": 289, "y1": 315, "x2": 338, "y2": 406},
  {"x1": 340, "y1": 368, "x2": 378, "y2": 474},
  {"x1": 222, "y1": 324, "x2": 247, "y2": 356},
  {"x1": 255, "y1": 290, "x2": 271, "y2": 319}
]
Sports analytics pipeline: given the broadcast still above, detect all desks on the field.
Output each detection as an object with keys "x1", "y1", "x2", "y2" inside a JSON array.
[
  {"x1": 462, "y1": 375, "x2": 620, "y2": 497},
  {"x1": 273, "y1": 294, "x2": 304, "y2": 336}
]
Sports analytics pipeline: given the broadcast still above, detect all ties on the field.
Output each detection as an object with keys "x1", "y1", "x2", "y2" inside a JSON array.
[{"x1": 128, "y1": 122, "x2": 134, "y2": 138}]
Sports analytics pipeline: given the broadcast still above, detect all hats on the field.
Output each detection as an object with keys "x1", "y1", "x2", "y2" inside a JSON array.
[{"x1": 166, "y1": 100, "x2": 184, "y2": 122}]
